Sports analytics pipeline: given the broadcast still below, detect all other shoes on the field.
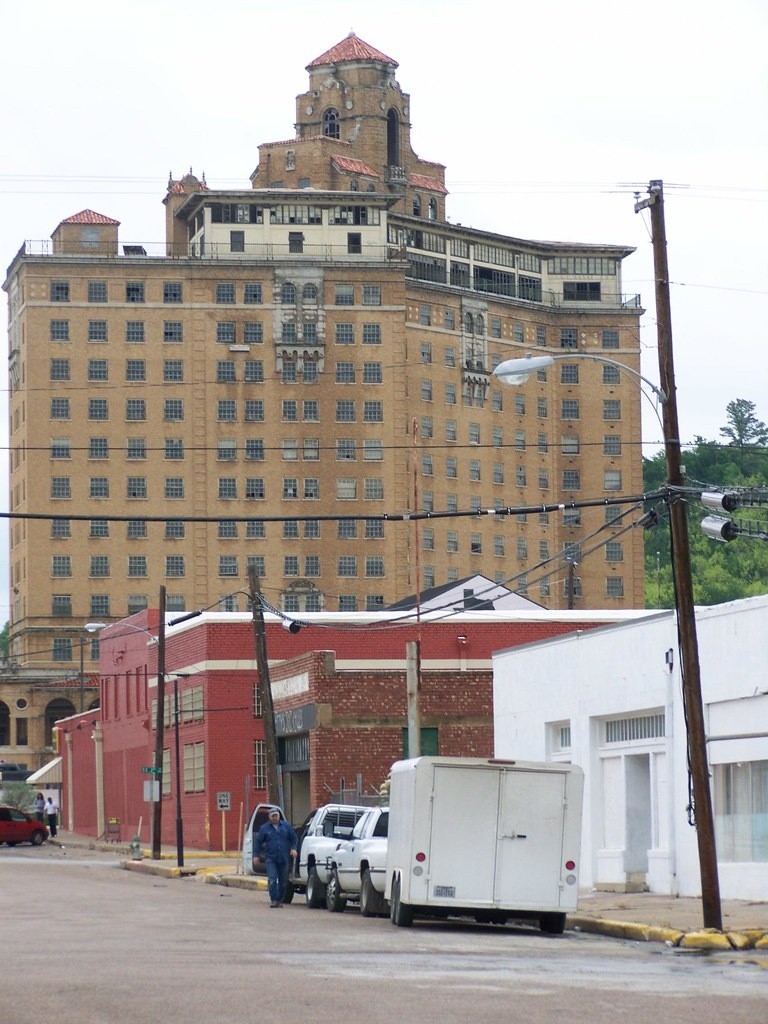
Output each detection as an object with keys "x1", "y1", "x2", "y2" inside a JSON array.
[
  {"x1": 50, "y1": 835, "x2": 56, "y2": 838},
  {"x1": 270, "y1": 900, "x2": 276, "y2": 907},
  {"x1": 277, "y1": 902, "x2": 283, "y2": 906}
]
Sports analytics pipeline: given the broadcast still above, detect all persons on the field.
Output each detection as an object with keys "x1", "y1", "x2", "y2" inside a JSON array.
[
  {"x1": 253, "y1": 808, "x2": 298, "y2": 907},
  {"x1": 34, "y1": 793, "x2": 45, "y2": 822},
  {"x1": 44, "y1": 797, "x2": 59, "y2": 837}
]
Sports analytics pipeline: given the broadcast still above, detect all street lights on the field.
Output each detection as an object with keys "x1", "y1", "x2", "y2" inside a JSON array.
[
  {"x1": 84, "y1": 624, "x2": 166, "y2": 860},
  {"x1": 491, "y1": 356, "x2": 723, "y2": 930}
]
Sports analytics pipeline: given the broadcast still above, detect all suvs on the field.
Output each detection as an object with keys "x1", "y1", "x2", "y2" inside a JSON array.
[
  {"x1": 326, "y1": 807, "x2": 389, "y2": 916},
  {"x1": 246, "y1": 804, "x2": 370, "y2": 909}
]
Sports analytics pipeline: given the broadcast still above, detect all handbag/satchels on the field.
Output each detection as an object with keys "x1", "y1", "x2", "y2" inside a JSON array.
[{"x1": 55, "y1": 808, "x2": 58, "y2": 816}]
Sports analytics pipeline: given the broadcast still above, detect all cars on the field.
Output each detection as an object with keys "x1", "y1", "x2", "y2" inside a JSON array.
[{"x1": 0, "y1": 805, "x2": 50, "y2": 846}]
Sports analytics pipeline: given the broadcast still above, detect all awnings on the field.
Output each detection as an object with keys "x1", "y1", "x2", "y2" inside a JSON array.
[
  {"x1": 26, "y1": 757, "x2": 62, "y2": 784},
  {"x1": 331, "y1": 154, "x2": 381, "y2": 183},
  {"x1": 409, "y1": 173, "x2": 449, "y2": 198}
]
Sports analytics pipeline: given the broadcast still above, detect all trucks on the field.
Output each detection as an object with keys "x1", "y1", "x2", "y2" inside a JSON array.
[{"x1": 383, "y1": 755, "x2": 586, "y2": 932}]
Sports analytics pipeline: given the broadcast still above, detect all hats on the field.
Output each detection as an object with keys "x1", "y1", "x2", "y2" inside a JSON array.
[{"x1": 269, "y1": 808, "x2": 279, "y2": 817}]
